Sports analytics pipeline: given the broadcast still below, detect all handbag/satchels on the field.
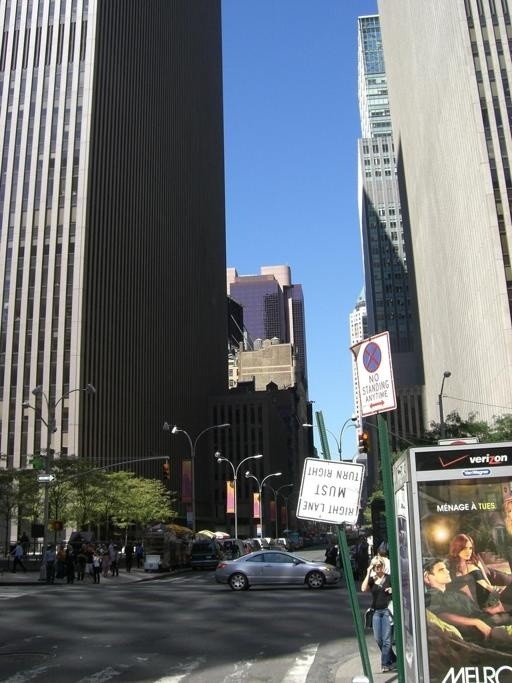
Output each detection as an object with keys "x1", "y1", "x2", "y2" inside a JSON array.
[{"x1": 365, "y1": 608, "x2": 374, "y2": 628}]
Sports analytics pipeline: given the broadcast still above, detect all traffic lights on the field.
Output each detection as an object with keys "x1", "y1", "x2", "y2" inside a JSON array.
[
  {"x1": 162, "y1": 464, "x2": 170, "y2": 481},
  {"x1": 359, "y1": 432, "x2": 369, "y2": 453},
  {"x1": 48, "y1": 520, "x2": 63, "y2": 532}
]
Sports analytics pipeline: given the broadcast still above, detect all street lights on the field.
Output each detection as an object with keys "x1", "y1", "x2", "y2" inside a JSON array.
[
  {"x1": 263, "y1": 482, "x2": 295, "y2": 537},
  {"x1": 163, "y1": 421, "x2": 231, "y2": 531},
  {"x1": 214, "y1": 452, "x2": 264, "y2": 538},
  {"x1": 438, "y1": 371, "x2": 451, "y2": 439},
  {"x1": 22, "y1": 382, "x2": 97, "y2": 581},
  {"x1": 244, "y1": 471, "x2": 282, "y2": 538},
  {"x1": 302, "y1": 417, "x2": 358, "y2": 461}
]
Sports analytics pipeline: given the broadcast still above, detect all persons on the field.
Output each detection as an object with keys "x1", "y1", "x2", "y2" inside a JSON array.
[
  {"x1": 444, "y1": 534, "x2": 512, "y2": 615},
  {"x1": 17, "y1": 531, "x2": 30, "y2": 559},
  {"x1": 423, "y1": 558, "x2": 512, "y2": 655},
  {"x1": 366, "y1": 547, "x2": 396, "y2": 646},
  {"x1": 377, "y1": 532, "x2": 389, "y2": 558},
  {"x1": 361, "y1": 553, "x2": 396, "y2": 672},
  {"x1": 355, "y1": 535, "x2": 369, "y2": 583},
  {"x1": 324, "y1": 542, "x2": 338, "y2": 566},
  {"x1": 45, "y1": 541, "x2": 145, "y2": 584},
  {"x1": 8, "y1": 541, "x2": 28, "y2": 573}
]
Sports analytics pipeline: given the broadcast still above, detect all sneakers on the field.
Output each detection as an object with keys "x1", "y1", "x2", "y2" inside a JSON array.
[{"x1": 383, "y1": 662, "x2": 396, "y2": 672}]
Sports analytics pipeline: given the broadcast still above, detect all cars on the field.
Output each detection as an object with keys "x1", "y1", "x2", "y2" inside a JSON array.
[
  {"x1": 190, "y1": 534, "x2": 329, "y2": 571},
  {"x1": 215, "y1": 550, "x2": 343, "y2": 591}
]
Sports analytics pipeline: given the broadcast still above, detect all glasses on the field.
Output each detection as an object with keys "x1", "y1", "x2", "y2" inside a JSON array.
[{"x1": 372, "y1": 564, "x2": 382, "y2": 568}]
